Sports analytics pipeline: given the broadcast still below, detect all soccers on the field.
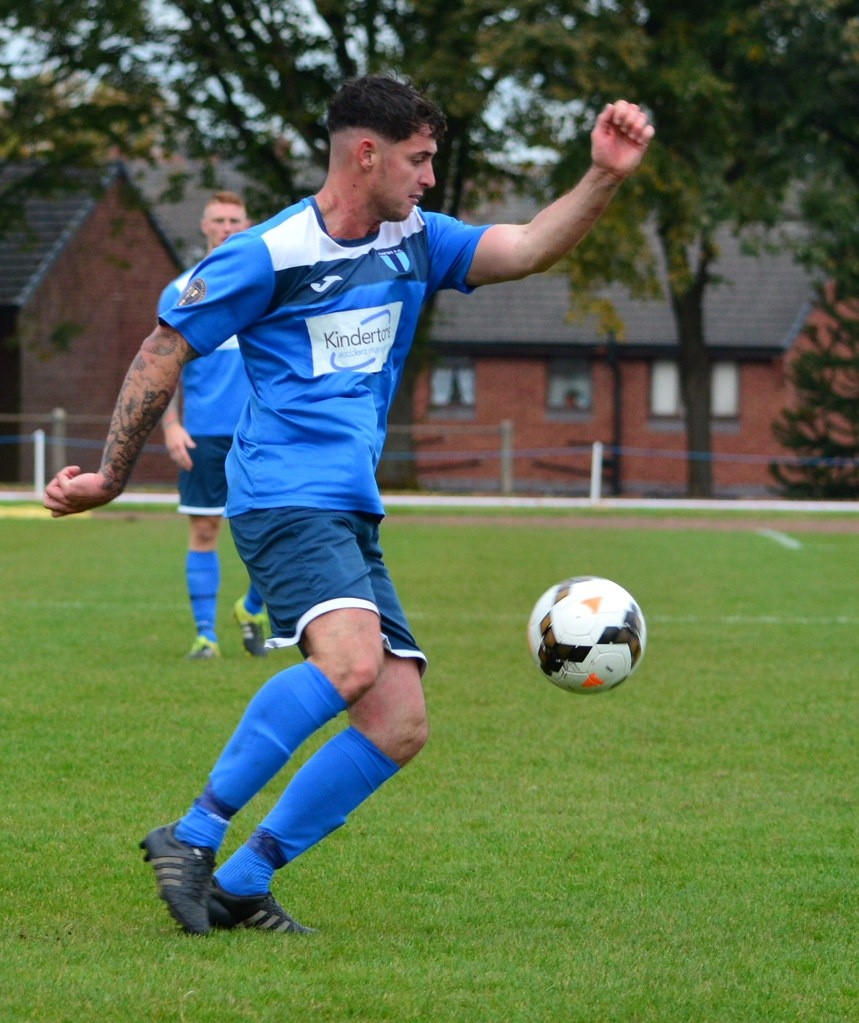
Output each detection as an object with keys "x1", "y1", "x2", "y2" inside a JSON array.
[{"x1": 528, "y1": 575, "x2": 650, "y2": 696}]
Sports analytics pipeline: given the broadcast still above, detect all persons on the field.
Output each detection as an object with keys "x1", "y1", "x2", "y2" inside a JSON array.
[
  {"x1": 42, "y1": 77, "x2": 655, "y2": 933},
  {"x1": 155, "y1": 191, "x2": 269, "y2": 659}
]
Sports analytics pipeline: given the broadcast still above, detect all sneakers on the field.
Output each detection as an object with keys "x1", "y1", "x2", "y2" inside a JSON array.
[
  {"x1": 139, "y1": 821, "x2": 219, "y2": 937},
  {"x1": 190, "y1": 634, "x2": 221, "y2": 661},
  {"x1": 205, "y1": 874, "x2": 320, "y2": 931},
  {"x1": 232, "y1": 593, "x2": 269, "y2": 657}
]
